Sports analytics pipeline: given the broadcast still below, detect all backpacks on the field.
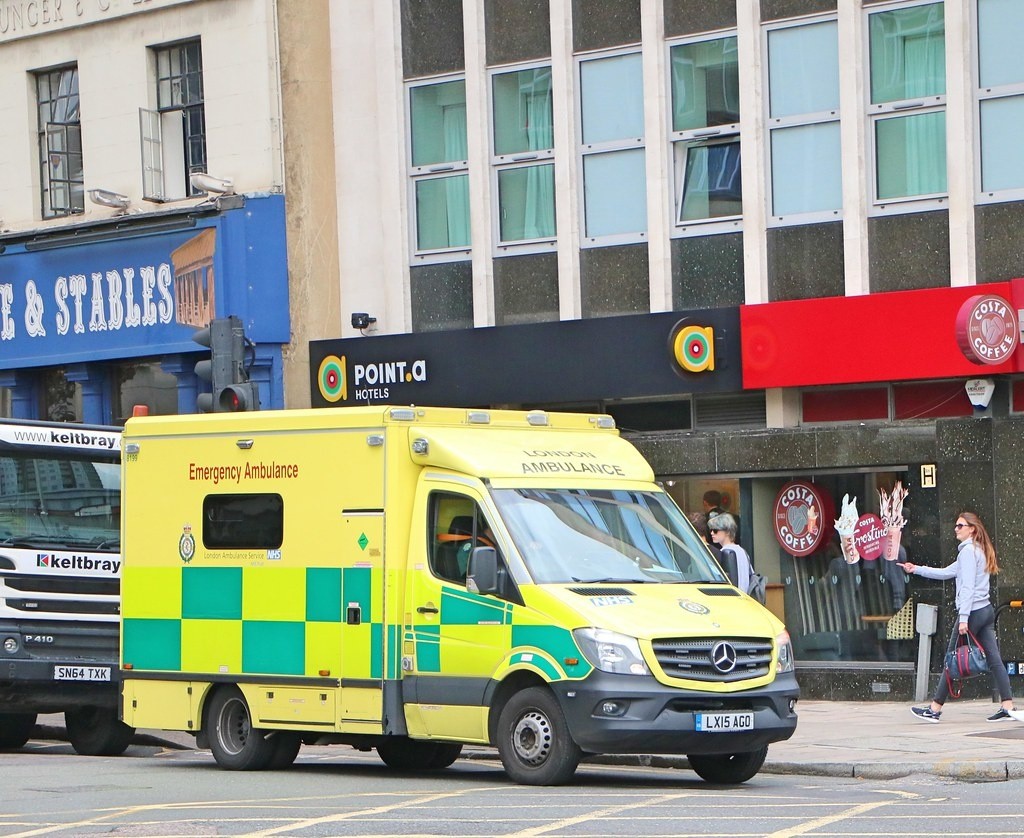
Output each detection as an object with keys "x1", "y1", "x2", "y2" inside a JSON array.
[{"x1": 744, "y1": 553, "x2": 768, "y2": 606}]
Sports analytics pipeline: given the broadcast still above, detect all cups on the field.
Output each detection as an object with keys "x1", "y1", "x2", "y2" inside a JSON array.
[
  {"x1": 840, "y1": 530, "x2": 860, "y2": 564},
  {"x1": 883, "y1": 527, "x2": 901, "y2": 561}
]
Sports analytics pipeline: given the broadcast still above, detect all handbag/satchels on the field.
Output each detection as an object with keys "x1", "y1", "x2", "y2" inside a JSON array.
[{"x1": 944, "y1": 629, "x2": 990, "y2": 698}]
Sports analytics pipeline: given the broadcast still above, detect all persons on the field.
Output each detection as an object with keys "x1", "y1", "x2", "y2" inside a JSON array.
[
  {"x1": 702, "y1": 490, "x2": 740, "y2": 549},
  {"x1": 455, "y1": 499, "x2": 543, "y2": 586},
  {"x1": 896, "y1": 512, "x2": 1016, "y2": 723},
  {"x1": 687, "y1": 513, "x2": 755, "y2": 594}
]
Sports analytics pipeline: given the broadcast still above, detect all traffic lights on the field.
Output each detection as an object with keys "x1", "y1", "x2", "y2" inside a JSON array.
[
  {"x1": 193, "y1": 314, "x2": 246, "y2": 413},
  {"x1": 219, "y1": 382, "x2": 260, "y2": 411}
]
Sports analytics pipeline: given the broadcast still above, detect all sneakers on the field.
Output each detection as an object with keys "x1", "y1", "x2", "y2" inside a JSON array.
[
  {"x1": 910, "y1": 705, "x2": 942, "y2": 724},
  {"x1": 986, "y1": 705, "x2": 1018, "y2": 723}
]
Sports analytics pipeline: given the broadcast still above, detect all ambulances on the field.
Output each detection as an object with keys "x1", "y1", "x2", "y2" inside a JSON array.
[{"x1": 119, "y1": 405, "x2": 799, "y2": 788}]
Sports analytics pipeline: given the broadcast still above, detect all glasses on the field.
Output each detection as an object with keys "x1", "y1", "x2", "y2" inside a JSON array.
[
  {"x1": 954, "y1": 523, "x2": 972, "y2": 530},
  {"x1": 708, "y1": 529, "x2": 725, "y2": 534}
]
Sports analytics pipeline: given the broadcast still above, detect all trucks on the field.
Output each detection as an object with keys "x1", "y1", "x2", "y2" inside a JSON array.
[{"x1": 1, "y1": 418, "x2": 136, "y2": 756}]
